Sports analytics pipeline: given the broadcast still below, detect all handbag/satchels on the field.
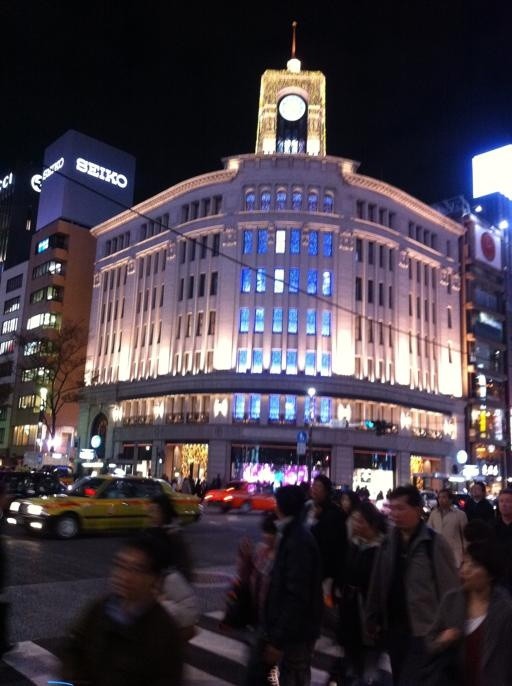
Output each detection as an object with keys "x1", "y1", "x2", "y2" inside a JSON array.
[{"x1": 219, "y1": 578, "x2": 259, "y2": 630}]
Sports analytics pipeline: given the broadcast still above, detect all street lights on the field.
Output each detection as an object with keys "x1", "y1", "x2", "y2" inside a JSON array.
[
  {"x1": 34, "y1": 386, "x2": 48, "y2": 452},
  {"x1": 307, "y1": 387, "x2": 317, "y2": 486}
]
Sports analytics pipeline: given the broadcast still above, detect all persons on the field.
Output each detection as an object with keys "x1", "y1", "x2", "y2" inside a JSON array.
[
  {"x1": 60, "y1": 531, "x2": 187, "y2": 686},
  {"x1": 68, "y1": 457, "x2": 77, "y2": 475},
  {"x1": 158, "y1": 472, "x2": 224, "y2": 503},
  {"x1": 126, "y1": 493, "x2": 201, "y2": 641},
  {"x1": 225, "y1": 470, "x2": 511, "y2": 684}
]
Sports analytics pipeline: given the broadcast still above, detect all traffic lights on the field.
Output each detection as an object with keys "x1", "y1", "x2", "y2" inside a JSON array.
[
  {"x1": 366, "y1": 419, "x2": 375, "y2": 430},
  {"x1": 468, "y1": 371, "x2": 483, "y2": 399}
]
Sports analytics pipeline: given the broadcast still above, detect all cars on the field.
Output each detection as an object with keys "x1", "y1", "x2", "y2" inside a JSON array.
[
  {"x1": 376, "y1": 487, "x2": 501, "y2": 521},
  {"x1": 1, "y1": 448, "x2": 280, "y2": 543}
]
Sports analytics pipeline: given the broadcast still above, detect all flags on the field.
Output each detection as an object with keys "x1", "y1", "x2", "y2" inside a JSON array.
[{"x1": 472, "y1": 223, "x2": 504, "y2": 273}]
